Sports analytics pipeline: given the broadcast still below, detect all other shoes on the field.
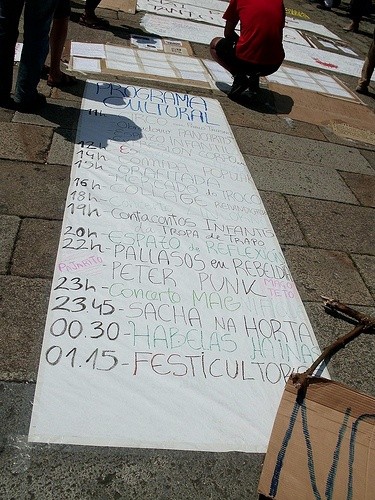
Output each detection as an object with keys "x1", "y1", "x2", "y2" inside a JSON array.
[
  {"x1": 343, "y1": 22, "x2": 358, "y2": 31},
  {"x1": 317, "y1": 4, "x2": 331, "y2": 11},
  {"x1": 43, "y1": 65, "x2": 50, "y2": 73},
  {"x1": 15, "y1": 93, "x2": 46, "y2": 108},
  {"x1": 356, "y1": 86, "x2": 368, "y2": 92},
  {"x1": 0, "y1": 98, "x2": 14, "y2": 108},
  {"x1": 47, "y1": 71, "x2": 76, "y2": 85},
  {"x1": 80, "y1": 17, "x2": 101, "y2": 27}
]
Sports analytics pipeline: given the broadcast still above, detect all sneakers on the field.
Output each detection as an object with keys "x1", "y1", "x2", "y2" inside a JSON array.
[
  {"x1": 228, "y1": 75, "x2": 250, "y2": 98},
  {"x1": 249, "y1": 75, "x2": 259, "y2": 92}
]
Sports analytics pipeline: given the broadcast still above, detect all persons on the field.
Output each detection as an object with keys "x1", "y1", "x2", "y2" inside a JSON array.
[
  {"x1": 1, "y1": 0, "x2": 49, "y2": 113},
  {"x1": 79, "y1": 0, "x2": 110, "y2": 30},
  {"x1": 209, "y1": 0, "x2": 286, "y2": 99},
  {"x1": 343, "y1": 0, "x2": 375, "y2": 32},
  {"x1": 316, "y1": 0, "x2": 342, "y2": 11},
  {"x1": 38, "y1": 0, "x2": 76, "y2": 87},
  {"x1": 355, "y1": 34, "x2": 375, "y2": 92}
]
